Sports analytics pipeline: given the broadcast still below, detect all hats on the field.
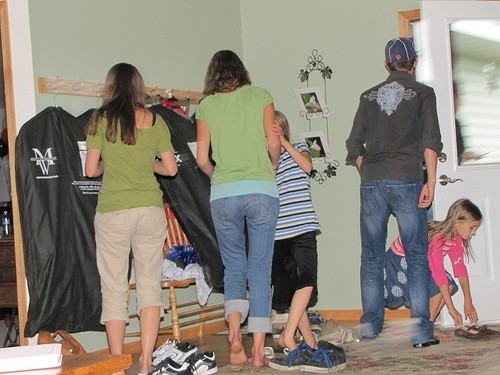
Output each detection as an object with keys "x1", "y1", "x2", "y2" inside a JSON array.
[{"x1": 384, "y1": 37, "x2": 416, "y2": 63}]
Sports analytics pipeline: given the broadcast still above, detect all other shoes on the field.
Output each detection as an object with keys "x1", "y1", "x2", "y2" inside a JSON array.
[
  {"x1": 307, "y1": 309, "x2": 329, "y2": 324},
  {"x1": 455, "y1": 324, "x2": 500, "y2": 342},
  {"x1": 413, "y1": 336, "x2": 440, "y2": 347},
  {"x1": 282, "y1": 324, "x2": 322, "y2": 341},
  {"x1": 361, "y1": 328, "x2": 383, "y2": 338}
]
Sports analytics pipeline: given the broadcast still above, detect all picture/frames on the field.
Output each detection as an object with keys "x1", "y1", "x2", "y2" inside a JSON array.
[
  {"x1": 294, "y1": 87, "x2": 327, "y2": 120},
  {"x1": 298, "y1": 131, "x2": 330, "y2": 162}
]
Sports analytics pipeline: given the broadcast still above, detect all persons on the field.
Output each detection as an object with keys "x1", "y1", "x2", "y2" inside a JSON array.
[
  {"x1": 381, "y1": 197, "x2": 484, "y2": 342},
  {"x1": 345, "y1": 37, "x2": 449, "y2": 348},
  {"x1": 85, "y1": 63, "x2": 179, "y2": 375},
  {"x1": 196, "y1": 48, "x2": 282, "y2": 369},
  {"x1": 263, "y1": 110, "x2": 323, "y2": 355}
]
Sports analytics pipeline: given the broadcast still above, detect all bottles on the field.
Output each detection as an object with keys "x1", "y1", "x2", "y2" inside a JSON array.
[{"x1": 2, "y1": 211, "x2": 11, "y2": 236}]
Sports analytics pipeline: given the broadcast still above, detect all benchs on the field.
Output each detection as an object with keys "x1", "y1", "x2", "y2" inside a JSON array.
[{"x1": 124, "y1": 204, "x2": 232, "y2": 346}]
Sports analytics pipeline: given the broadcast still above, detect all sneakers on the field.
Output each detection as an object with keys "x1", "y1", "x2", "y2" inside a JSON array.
[
  {"x1": 303, "y1": 349, "x2": 346, "y2": 373},
  {"x1": 152, "y1": 338, "x2": 198, "y2": 367},
  {"x1": 268, "y1": 343, "x2": 316, "y2": 371},
  {"x1": 146, "y1": 351, "x2": 218, "y2": 375}
]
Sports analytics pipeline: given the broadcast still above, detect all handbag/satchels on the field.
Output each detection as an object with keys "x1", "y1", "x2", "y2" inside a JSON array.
[{"x1": 38, "y1": 330, "x2": 89, "y2": 356}]
